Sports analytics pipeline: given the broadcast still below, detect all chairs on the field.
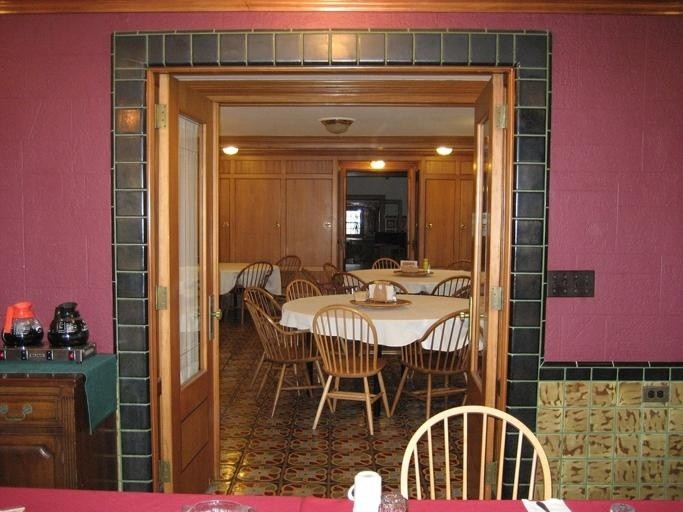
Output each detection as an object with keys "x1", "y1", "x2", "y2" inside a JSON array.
[
  {"x1": 324, "y1": 262, "x2": 348, "y2": 281},
  {"x1": 0, "y1": 486, "x2": 683, "y2": 512},
  {"x1": 243, "y1": 287, "x2": 316, "y2": 401},
  {"x1": 243, "y1": 299, "x2": 334, "y2": 419},
  {"x1": 445, "y1": 259, "x2": 474, "y2": 271},
  {"x1": 411, "y1": 284, "x2": 485, "y2": 385},
  {"x1": 331, "y1": 273, "x2": 365, "y2": 294},
  {"x1": 390, "y1": 309, "x2": 472, "y2": 422},
  {"x1": 301, "y1": 268, "x2": 337, "y2": 296},
  {"x1": 276, "y1": 255, "x2": 302, "y2": 290},
  {"x1": 313, "y1": 304, "x2": 391, "y2": 437},
  {"x1": 360, "y1": 280, "x2": 409, "y2": 380},
  {"x1": 225, "y1": 261, "x2": 274, "y2": 326},
  {"x1": 371, "y1": 258, "x2": 399, "y2": 268},
  {"x1": 400, "y1": 404, "x2": 551, "y2": 500},
  {"x1": 281, "y1": 278, "x2": 323, "y2": 383},
  {"x1": 430, "y1": 276, "x2": 471, "y2": 296}
]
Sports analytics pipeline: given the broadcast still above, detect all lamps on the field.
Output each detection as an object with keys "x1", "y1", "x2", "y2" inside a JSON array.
[
  {"x1": 223, "y1": 147, "x2": 239, "y2": 156},
  {"x1": 320, "y1": 118, "x2": 353, "y2": 134},
  {"x1": 436, "y1": 147, "x2": 452, "y2": 156}
]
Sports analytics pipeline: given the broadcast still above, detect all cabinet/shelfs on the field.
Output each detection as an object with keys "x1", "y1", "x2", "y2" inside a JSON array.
[{"x1": 0, "y1": 353, "x2": 121, "y2": 492}]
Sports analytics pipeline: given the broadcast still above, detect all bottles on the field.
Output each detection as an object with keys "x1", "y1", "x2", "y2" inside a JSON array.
[{"x1": 423, "y1": 259, "x2": 429, "y2": 272}]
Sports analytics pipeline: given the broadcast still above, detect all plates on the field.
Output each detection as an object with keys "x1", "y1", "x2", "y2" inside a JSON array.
[{"x1": 180, "y1": 500, "x2": 253, "y2": 512}]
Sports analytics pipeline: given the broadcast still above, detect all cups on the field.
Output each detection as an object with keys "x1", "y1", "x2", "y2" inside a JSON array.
[
  {"x1": 377, "y1": 492, "x2": 409, "y2": 512},
  {"x1": 346, "y1": 470, "x2": 381, "y2": 512}
]
